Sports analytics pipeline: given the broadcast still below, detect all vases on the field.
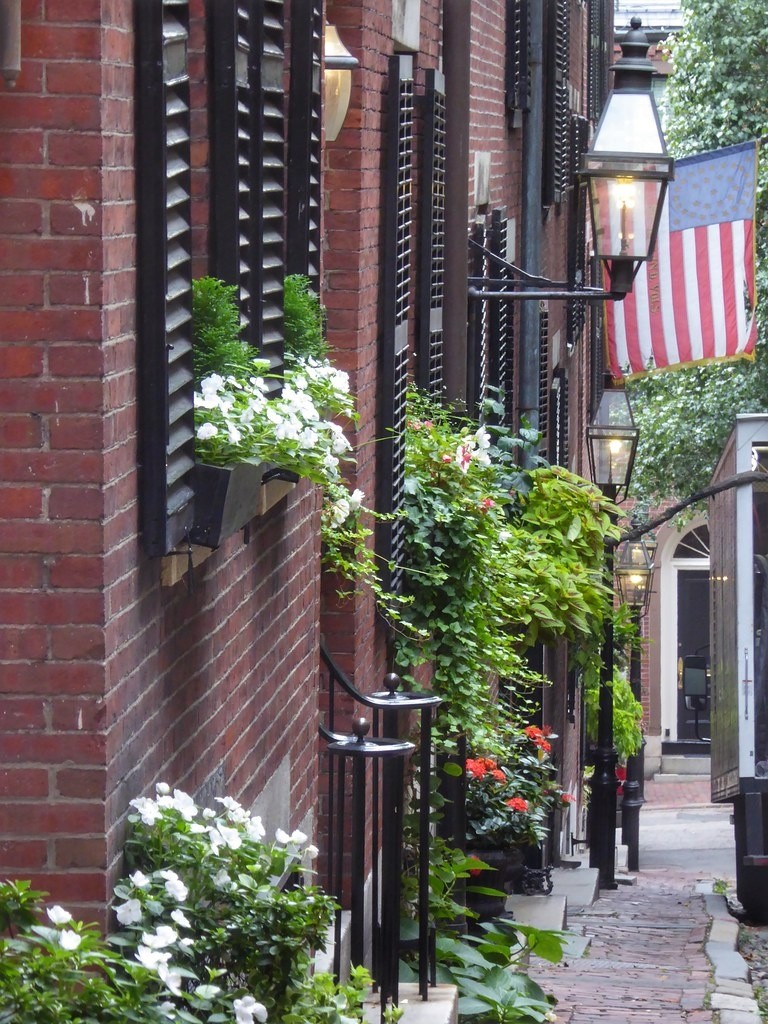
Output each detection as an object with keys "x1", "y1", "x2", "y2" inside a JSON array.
[{"x1": 467, "y1": 846, "x2": 517, "y2": 931}]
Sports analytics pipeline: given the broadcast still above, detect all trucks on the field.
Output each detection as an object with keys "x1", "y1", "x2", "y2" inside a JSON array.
[{"x1": 681, "y1": 414, "x2": 768, "y2": 923}]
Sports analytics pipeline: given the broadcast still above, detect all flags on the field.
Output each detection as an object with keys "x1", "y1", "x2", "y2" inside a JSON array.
[{"x1": 586, "y1": 142, "x2": 760, "y2": 385}]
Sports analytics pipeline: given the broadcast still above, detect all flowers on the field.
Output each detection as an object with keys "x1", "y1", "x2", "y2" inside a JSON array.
[
  {"x1": 0, "y1": 782, "x2": 318, "y2": 1024},
  {"x1": 465, "y1": 724, "x2": 574, "y2": 851},
  {"x1": 192, "y1": 359, "x2": 515, "y2": 637}
]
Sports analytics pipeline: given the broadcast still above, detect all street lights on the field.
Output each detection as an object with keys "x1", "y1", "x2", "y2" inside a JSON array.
[
  {"x1": 583, "y1": 385, "x2": 640, "y2": 890},
  {"x1": 615, "y1": 513, "x2": 656, "y2": 842}
]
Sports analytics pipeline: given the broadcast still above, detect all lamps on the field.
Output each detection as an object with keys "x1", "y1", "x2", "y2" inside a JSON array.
[
  {"x1": 467, "y1": 16, "x2": 675, "y2": 304},
  {"x1": 322, "y1": 20, "x2": 358, "y2": 142}
]
